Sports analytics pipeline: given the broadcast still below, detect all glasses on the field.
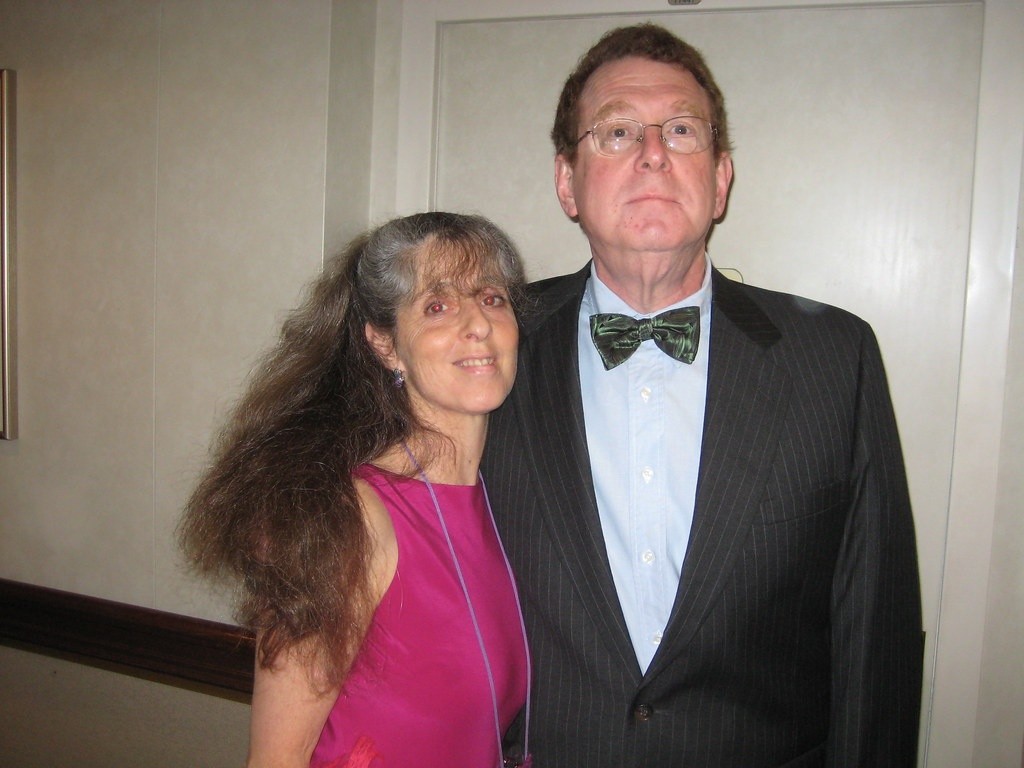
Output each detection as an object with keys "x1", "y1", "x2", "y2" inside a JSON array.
[{"x1": 556, "y1": 116, "x2": 719, "y2": 157}]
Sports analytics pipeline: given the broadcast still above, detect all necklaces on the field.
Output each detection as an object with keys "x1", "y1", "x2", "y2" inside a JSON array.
[{"x1": 405, "y1": 443, "x2": 531, "y2": 768}]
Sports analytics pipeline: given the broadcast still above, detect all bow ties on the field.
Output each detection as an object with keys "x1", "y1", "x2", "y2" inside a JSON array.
[{"x1": 588, "y1": 307, "x2": 700, "y2": 372}]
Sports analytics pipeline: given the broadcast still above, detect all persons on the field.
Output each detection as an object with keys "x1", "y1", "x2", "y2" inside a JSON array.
[
  {"x1": 480, "y1": 23, "x2": 924, "y2": 768},
  {"x1": 179, "y1": 212, "x2": 532, "y2": 768}
]
